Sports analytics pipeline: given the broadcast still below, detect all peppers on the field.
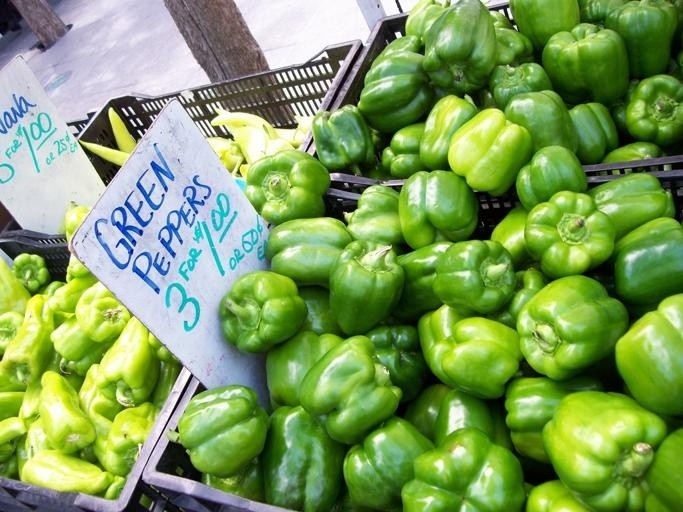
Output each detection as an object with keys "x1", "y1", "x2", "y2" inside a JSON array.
[{"x1": 1, "y1": 0, "x2": 682, "y2": 512}]
